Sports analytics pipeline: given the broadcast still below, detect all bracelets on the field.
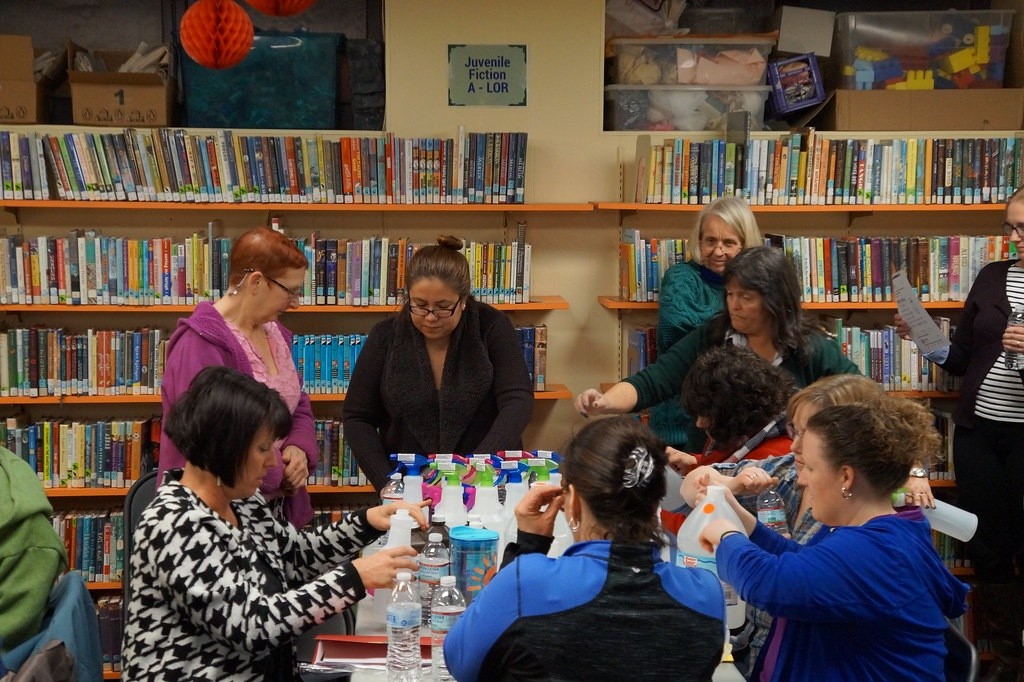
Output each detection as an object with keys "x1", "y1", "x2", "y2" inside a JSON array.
[{"x1": 720, "y1": 531, "x2": 746, "y2": 543}]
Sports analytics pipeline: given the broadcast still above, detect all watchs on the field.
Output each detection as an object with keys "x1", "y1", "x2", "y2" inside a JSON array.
[{"x1": 908, "y1": 467, "x2": 928, "y2": 478}]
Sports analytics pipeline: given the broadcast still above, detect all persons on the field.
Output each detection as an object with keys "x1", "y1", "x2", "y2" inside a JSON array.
[
  {"x1": 894, "y1": 185, "x2": 1024, "y2": 664},
  {"x1": 0, "y1": 446, "x2": 69, "y2": 660},
  {"x1": 647, "y1": 196, "x2": 764, "y2": 453},
  {"x1": 341, "y1": 234, "x2": 534, "y2": 502},
  {"x1": 121, "y1": 366, "x2": 431, "y2": 682},
  {"x1": 573, "y1": 246, "x2": 867, "y2": 419},
  {"x1": 659, "y1": 342, "x2": 800, "y2": 537},
  {"x1": 679, "y1": 373, "x2": 970, "y2": 682},
  {"x1": 157, "y1": 227, "x2": 319, "y2": 532},
  {"x1": 443, "y1": 417, "x2": 727, "y2": 682}
]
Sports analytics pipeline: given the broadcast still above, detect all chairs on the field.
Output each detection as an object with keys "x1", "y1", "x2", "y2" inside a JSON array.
[
  {"x1": 122, "y1": 469, "x2": 157, "y2": 623},
  {"x1": 941, "y1": 616, "x2": 977, "y2": 682}
]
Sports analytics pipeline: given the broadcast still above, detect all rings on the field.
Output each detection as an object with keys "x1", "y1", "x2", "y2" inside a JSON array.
[
  {"x1": 920, "y1": 492, "x2": 926, "y2": 495},
  {"x1": 750, "y1": 474, "x2": 756, "y2": 479},
  {"x1": 915, "y1": 494, "x2": 921, "y2": 496},
  {"x1": 1017, "y1": 340, "x2": 1020, "y2": 346},
  {"x1": 578, "y1": 395, "x2": 581, "y2": 397},
  {"x1": 906, "y1": 493, "x2": 914, "y2": 498}
]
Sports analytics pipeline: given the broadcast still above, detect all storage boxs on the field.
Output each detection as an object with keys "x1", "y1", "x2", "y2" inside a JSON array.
[
  {"x1": 679, "y1": 8, "x2": 744, "y2": 34},
  {"x1": 172, "y1": 31, "x2": 345, "y2": 129},
  {"x1": 609, "y1": 37, "x2": 777, "y2": 87},
  {"x1": 605, "y1": 84, "x2": 774, "y2": 131},
  {"x1": 829, "y1": 10, "x2": 1016, "y2": 89},
  {"x1": 68, "y1": 37, "x2": 175, "y2": 126},
  {"x1": 770, "y1": 6, "x2": 837, "y2": 57},
  {"x1": 798, "y1": 89, "x2": 1024, "y2": 132},
  {"x1": 0, "y1": 34, "x2": 72, "y2": 124},
  {"x1": 767, "y1": 52, "x2": 825, "y2": 113}
]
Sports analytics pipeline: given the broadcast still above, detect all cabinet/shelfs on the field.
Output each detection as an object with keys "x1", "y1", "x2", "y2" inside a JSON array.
[
  {"x1": 591, "y1": 200, "x2": 1024, "y2": 658},
  {"x1": 0, "y1": 198, "x2": 593, "y2": 682}
]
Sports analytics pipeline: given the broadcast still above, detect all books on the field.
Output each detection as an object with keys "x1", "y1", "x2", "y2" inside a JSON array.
[
  {"x1": 0, "y1": 128, "x2": 548, "y2": 673},
  {"x1": 618, "y1": 110, "x2": 1024, "y2": 653}
]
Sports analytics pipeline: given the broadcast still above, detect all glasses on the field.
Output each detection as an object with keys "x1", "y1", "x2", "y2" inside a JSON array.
[
  {"x1": 244, "y1": 269, "x2": 304, "y2": 299},
  {"x1": 407, "y1": 297, "x2": 460, "y2": 318},
  {"x1": 1002, "y1": 222, "x2": 1024, "y2": 237},
  {"x1": 786, "y1": 422, "x2": 804, "y2": 441},
  {"x1": 550, "y1": 481, "x2": 570, "y2": 512},
  {"x1": 699, "y1": 239, "x2": 742, "y2": 253}
]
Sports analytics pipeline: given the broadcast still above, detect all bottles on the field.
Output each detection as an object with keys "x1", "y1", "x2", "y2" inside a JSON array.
[
  {"x1": 1005, "y1": 305, "x2": 1024, "y2": 370},
  {"x1": 430, "y1": 577, "x2": 467, "y2": 682},
  {"x1": 659, "y1": 465, "x2": 694, "y2": 518},
  {"x1": 381, "y1": 472, "x2": 404, "y2": 506},
  {"x1": 383, "y1": 572, "x2": 423, "y2": 682},
  {"x1": 675, "y1": 486, "x2": 749, "y2": 630},
  {"x1": 424, "y1": 514, "x2": 489, "y2": 550},
  {"x1": 756, "y1": 486, "x2": 791, "y2": 539},
  {"x1": 890, "y1": 488, "x2": 980, "y2": 543},
  {"x1": 419, "y1": 532, "x2": 450, "y2": 631}
]
